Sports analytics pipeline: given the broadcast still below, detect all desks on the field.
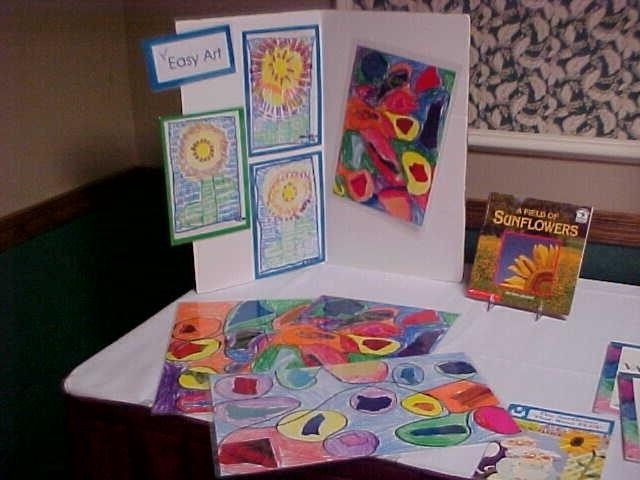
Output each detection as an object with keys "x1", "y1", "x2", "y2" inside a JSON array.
[{"x1": 63, "y1": 263, "x2": 640, "y2": 480}]
